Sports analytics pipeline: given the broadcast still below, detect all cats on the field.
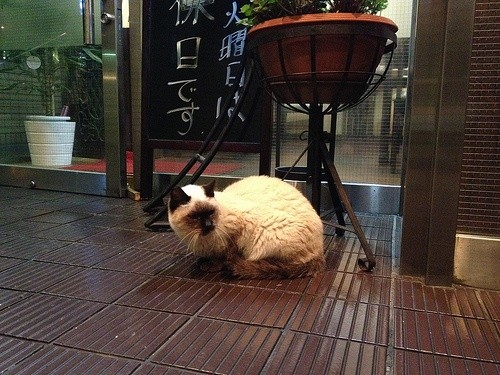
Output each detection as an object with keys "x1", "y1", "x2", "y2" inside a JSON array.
[{"x1": 168, "y1": 176, "x2": 323, "y2": 278}]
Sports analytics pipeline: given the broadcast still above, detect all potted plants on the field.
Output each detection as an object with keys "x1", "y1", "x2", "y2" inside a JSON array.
[
  {"x1": 242, "y1": 0, "x2": 398, "y2": 105},
  {"x1": 0, "y1": 46, "x2": 102, "y2": 167}
]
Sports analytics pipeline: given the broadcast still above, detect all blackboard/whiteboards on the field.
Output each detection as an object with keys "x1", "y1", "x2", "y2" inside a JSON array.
[{"x1": 141, "y1": 1, "x2": 274, "y2": 201}]
